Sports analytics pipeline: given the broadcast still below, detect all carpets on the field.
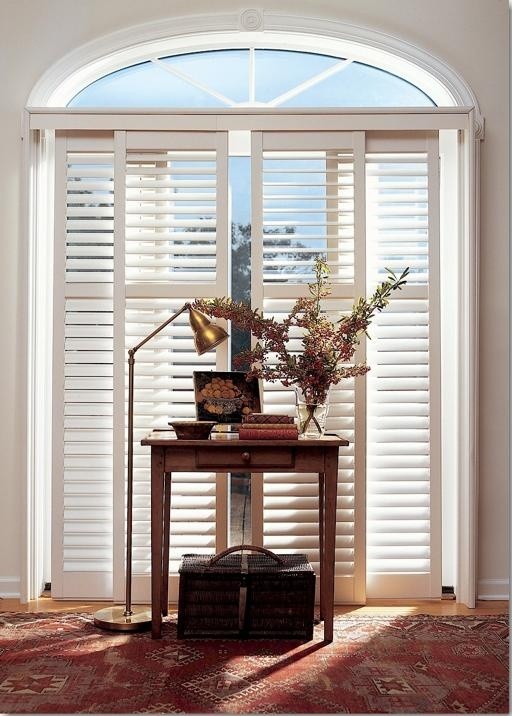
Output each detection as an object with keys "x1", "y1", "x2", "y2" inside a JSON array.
[{"x1": 2, "y1": 607, "x2": 510, "y2": 714}]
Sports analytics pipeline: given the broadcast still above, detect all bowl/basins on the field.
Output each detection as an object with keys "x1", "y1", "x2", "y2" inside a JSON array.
[{"x1": 168, "y1": 421, "x2": 218, "y2": 440}]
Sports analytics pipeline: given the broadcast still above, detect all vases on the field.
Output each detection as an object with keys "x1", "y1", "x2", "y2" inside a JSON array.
[{"x1": 293, "y1": 384, "x2": 330, "y2": 439}]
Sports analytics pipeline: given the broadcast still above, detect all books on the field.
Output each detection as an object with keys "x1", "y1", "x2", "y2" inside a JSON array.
[
  {"x1": 242, "y1": 424, "x2": 297, "y2": 429},
  {"x1": 240, "y1": 414, "x2": 295, "y2": 424},
  {"x1": 239, "y1": 429, "x2": 299, "y2": 440}
]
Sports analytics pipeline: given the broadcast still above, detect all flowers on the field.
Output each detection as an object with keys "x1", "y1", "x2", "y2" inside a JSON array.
[{"x1": 192, "y1": 251, "x2": 411, "y2": 430}]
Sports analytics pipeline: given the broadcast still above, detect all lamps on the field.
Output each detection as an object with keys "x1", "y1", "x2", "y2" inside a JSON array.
[{"x1": 91, "y1": 299, "x2": 230, "y2": 633}]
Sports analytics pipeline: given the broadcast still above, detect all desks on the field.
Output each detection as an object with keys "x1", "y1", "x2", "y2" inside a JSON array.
[{"x1": 140, "y1": 427, "x2": 349, "y2": 640}]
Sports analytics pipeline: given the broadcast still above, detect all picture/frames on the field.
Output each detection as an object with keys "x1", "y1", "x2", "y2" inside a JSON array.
[{"x1": 193, "y1": 370, "x2": 264, "y2": 428}]
